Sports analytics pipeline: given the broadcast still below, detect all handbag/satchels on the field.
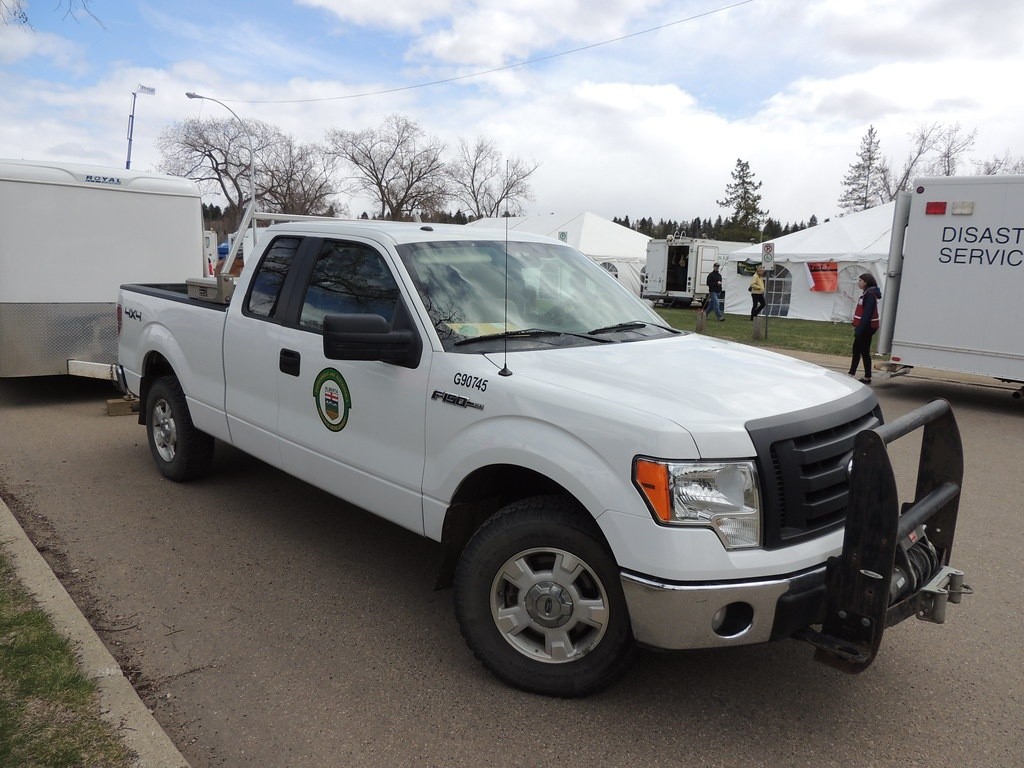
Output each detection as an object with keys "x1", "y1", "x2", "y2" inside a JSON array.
[{"x1": 748, "y1": 286, "x2": 752, "y2": 292}]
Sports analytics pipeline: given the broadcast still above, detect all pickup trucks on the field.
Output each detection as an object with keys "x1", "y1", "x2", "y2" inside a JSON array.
[{"x1": 116, "y1": 213, "x2": 975, "y2": 698}]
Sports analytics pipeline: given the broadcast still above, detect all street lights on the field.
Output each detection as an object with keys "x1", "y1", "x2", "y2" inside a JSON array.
[{"x1": 185, "y1": 90, "x2": 258, "y2": 249}]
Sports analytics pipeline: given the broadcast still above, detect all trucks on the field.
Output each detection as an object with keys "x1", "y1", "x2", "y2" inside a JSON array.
[
  {"x1": 872, "y1": 175, "x2": 1022, "y2": 400},
  {"x1": 643, "y1": 238, "x2": 758, "y2": 310}
]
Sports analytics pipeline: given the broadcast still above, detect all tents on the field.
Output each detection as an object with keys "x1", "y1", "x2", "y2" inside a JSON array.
[
  {"x1": 465, "y1": 211, "x2": 654, "y2": 299},
  {"x1": 723, "y1": 201, "x2": 896, "y2": 324}
]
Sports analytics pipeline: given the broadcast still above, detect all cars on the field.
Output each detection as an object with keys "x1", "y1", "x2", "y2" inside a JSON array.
[{"x1": 204, "y1": 229, "x2": 243, "y2": 277}]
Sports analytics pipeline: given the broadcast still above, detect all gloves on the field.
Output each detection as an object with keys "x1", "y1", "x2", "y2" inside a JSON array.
[{"x1": 718, "y1": 281, "x2": 722, "y2": 285}]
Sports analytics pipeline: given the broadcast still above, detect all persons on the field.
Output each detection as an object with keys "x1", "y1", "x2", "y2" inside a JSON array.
[
  {"x1": 705, "y1": 263, "x2": 725, "y2": 322},
  {"x1": 848, "y1": 274, "x2": 882, "y2": 384},
  {"x1": 751, "y1": 266, "x2": 766, "y2": 321}
]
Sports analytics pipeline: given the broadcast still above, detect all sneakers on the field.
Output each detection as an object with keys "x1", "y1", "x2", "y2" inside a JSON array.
[
  {"x1": 706, "y1": 314, "x2": 711, "y2": 320},
  {"x1": 719, "y1": 317, "x2": 725, "y2": 321}
]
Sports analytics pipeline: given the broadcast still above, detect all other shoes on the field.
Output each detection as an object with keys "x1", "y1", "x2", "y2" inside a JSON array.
[{"x1": 858, "y1": 377, "x2": 872, "y2": 384}]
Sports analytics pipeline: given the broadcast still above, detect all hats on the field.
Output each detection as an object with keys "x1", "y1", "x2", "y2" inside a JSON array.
[{"x1": 714, "y1": 263, "x2": 720, "y2": 266}]
